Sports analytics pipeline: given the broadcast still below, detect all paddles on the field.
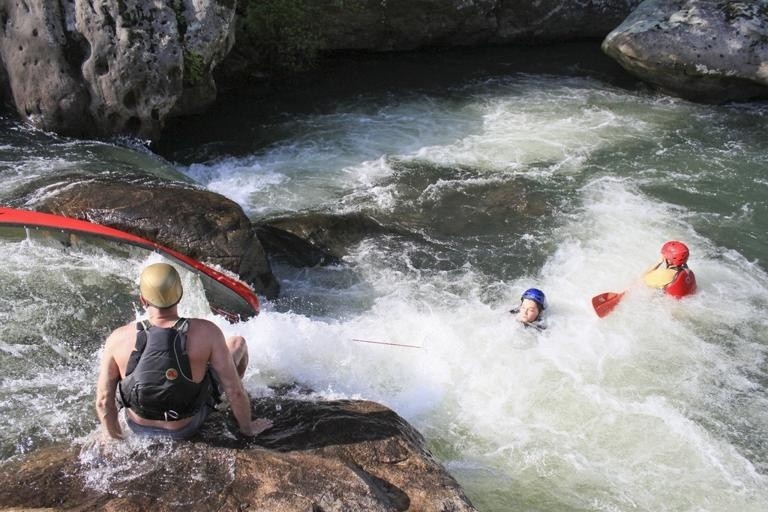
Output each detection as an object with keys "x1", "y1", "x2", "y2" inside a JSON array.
[{"x1": 592, "y1": 259, "x2": 663, "y2": 319}]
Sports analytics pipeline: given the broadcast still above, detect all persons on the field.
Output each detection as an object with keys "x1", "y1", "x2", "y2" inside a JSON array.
[
  {"x1": 516, "y1": 288, "x2": 549, "y2": 324},
  {"x1": 95, "y1": 262, "x2": 275, "y2": 445},
  {"x1": 659, "y1": 240, "x2": 697, "y2": 300}
]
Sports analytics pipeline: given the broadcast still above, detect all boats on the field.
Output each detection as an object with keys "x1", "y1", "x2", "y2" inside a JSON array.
[{"x1": 0, "y1": 207, "x2": 260, "y2": 324}]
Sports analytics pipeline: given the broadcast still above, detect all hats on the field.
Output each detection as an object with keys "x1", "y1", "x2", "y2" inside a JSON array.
[{"x1": 138, "y1": 262, "x2": 184, "y2": 309}]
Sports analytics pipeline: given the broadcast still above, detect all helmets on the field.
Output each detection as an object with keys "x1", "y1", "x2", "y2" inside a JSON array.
[
  {"x1": 660, "y1": 240, "x2": 690, "y2": 268},
  {"x1": 520, "y1": 287, "x2": 547, "y2": 310}
]
